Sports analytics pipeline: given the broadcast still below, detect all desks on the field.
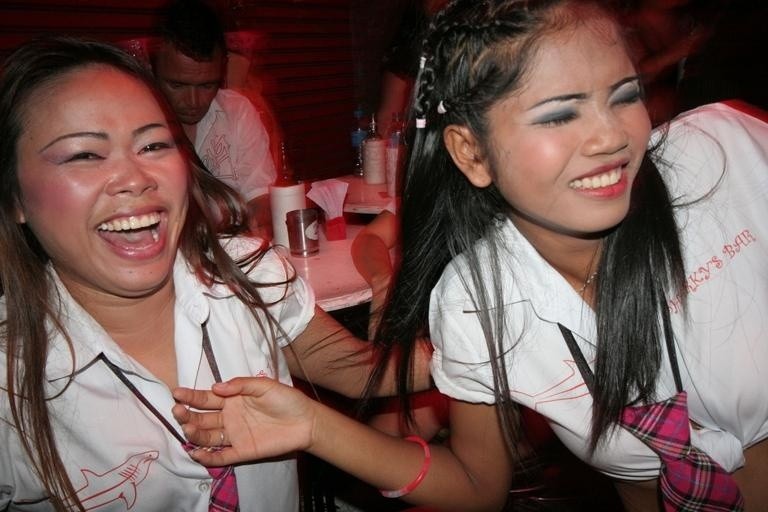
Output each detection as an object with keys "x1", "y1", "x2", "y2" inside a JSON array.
[
  {"x1": 310, "y1": 168, "x2": 405, "y2": 215},
  {"x1": 251, "y1": 222, "x2": 401, "y2": 315}
]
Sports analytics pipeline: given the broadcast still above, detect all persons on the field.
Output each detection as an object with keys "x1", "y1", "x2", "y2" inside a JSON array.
[
  {"x1": 0, "y1": 40, "x2": 436, "y2": 512},
  {"x1": 375, "y1": 1, "x2": 458, "y2": 143},
  {"x1": 167, "y1": 2, "x2": 767, "y2": 512},
  {"x1": 146, "y1": 14, "x2": 279, "y2": 233}
]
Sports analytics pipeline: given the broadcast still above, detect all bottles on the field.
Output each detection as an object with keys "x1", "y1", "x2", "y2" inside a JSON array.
[
  {"x1": 351, "y1": 110, "x2": 367, "y2": 177},
  {"x1": 365, "y1": 113, "x2": 383, "y2": 140},
  {"x1": 272, "y1": 141, "x2": 297, "y2": 187},
  {"x1": 385, "y1": 110, "x2": 402, "y2": 146}
]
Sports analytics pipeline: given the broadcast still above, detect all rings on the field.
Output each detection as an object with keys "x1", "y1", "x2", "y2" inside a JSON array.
[{"x1": 219, "y1": 431, "x2": 224, "y2": 446}]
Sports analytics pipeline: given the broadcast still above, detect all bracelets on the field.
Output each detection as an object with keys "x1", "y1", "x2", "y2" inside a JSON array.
[{"x1": 380, "y1": 434, "x2": 432, "y2": 498}]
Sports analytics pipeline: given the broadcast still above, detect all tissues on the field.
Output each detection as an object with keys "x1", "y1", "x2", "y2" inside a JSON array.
[{"x1": 305, "y1": 177, "x2": 349, "y2": 241}]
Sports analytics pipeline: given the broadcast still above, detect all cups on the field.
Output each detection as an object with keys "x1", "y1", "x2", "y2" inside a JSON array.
[{"x1": 283, "y1": 208, "x2": 320, "y2": 258}]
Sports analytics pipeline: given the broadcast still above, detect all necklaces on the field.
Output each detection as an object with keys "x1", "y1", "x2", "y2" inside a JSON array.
[{"x1": 571, "y1": 253, "x2": 601, "y2": 296}]
